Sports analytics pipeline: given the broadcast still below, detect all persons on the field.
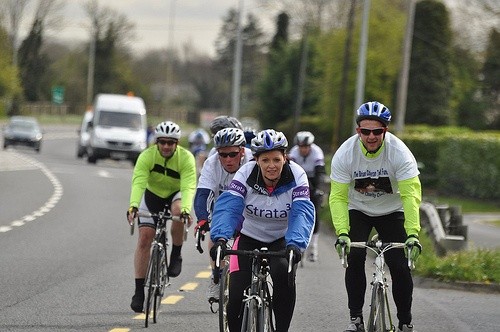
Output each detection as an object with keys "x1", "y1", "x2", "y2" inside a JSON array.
[
  {"x1": 329, "y1": 101, "x2": 422, "y2": 332},
  {"x1": 210, "y1": 129, "x2": 316, "y2": 332},
  {"x1": 127, "y1": 121, "x2": 196, "y2": 312},
  {"x1": 188, "y1": 118, "x2": 255, "y2": 280},
  {"x1": 288, "y1": 131, "x2": 325, "y2": 261}
]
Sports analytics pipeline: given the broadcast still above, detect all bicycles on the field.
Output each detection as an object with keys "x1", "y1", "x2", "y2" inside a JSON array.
[
  {"x1": 340, "y1": 240, "x2": 420, "y2": 331},
  {"x1": 197, "y1": 215, "x2": 298, "y2": 331},
  {"x1": 131, "y1": 211, "x2": 190, "y2": 327}
]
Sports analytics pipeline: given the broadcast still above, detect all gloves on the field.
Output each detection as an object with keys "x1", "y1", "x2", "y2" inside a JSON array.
[
  {"x1": 335, "y1": 233, "x2": 350, "y2": 257},
  {"x1": 194, "y1": 221, "x2": 210, "y2": 238},
  {"x1": 286, "y1": 245, "x2": 300, "y2": 264},
  {"x1": 210, "y1": 240, "x2": 227, "y2": 261},
  {"x1": 404, "y1": 235, "x2": 422, "y2": 261}
]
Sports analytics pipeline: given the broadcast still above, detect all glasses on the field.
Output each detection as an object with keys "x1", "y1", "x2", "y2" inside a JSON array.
[
  {"x1": 157, "y1": 140, "x2": 177, "y2": 145},
  {"x1": 219, "y1": 152, "x2": 239, "y2": 157},
  {"x1": 360, "y1": 129, "x2": 384, "y2": 135}
]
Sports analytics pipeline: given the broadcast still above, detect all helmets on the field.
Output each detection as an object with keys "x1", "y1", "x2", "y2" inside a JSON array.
[
  {"x1": 214, "y1": 128, "x2": 246, "y2": 147},
  {"x1": 188, "y1": 129, "x2": 211, "y2": 152},
  {"x1": 251, "y1": 129, "x2": 288, "y2": 156},
  {"x1": 244, "y1": 128, "x2": 256, "y2": 148},
  {"x1": 210, "y1": 116, "x2": 243, "y2": 134},
  {"x1": 294, "y1": 131, "x2": 314, "y2": 145},
  {"x1": 155, "y1": 121, "x2": 181, "y2": 139},
  {"x1": 356, "y1": 101, "x2": 391, "y2": 127}
]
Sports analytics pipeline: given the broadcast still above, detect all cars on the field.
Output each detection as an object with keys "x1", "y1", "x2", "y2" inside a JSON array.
[
  {"x1": 4, "y1": 116, "x2": 43, "y2": 152},
  {"x1": 78, "y1": 92, "x2": 150, "y2": 164}
]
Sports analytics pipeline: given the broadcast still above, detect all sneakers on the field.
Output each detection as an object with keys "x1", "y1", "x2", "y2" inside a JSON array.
[
  {"x1": 396, "y1": 324, "x2": 413, "y2": 332},
  {"x1": 345, "y1": 317, "x2": 365, "y2": 332}
]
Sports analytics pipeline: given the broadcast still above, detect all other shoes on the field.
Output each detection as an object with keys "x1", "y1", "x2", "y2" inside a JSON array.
[
  {"x1": 168, "y1": 254, "x2": 182, "y2": 276},
  {"x1": 208, "y1": 275, "x2": 220, "y2": 299},
  {"x1": 131, "y1": 291, "x2": 144, "y2": 313},
  {"x1": 308, "y1": 248, "x2": 317, "y2": 261}
]
